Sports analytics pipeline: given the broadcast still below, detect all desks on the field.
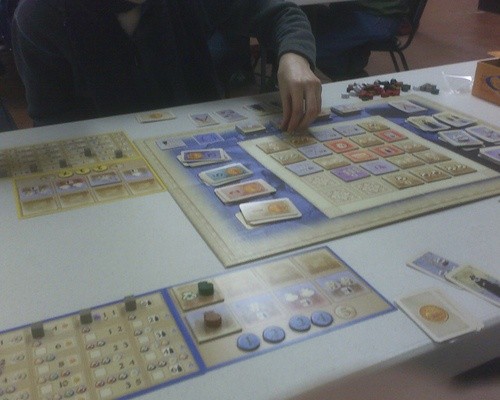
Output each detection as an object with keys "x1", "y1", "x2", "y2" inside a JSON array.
[{"x1": 0, "y1": 56, "x2": 500, "y2": 399}]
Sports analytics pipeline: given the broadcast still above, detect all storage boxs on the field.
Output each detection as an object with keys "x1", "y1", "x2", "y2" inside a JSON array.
[{"x1": 472, "y1": 59, "x2": 500, "y2": 104}]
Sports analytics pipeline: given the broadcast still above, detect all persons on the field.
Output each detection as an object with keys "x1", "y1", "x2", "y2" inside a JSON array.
[
  {"x1": 299, "y1": 0, "x2": 420, "y2": 82},
  {"x1": 10, "y1": 0, "x2": 323, "y2": 134}
]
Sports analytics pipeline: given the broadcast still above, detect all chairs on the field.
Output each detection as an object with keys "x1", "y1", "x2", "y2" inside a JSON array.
[{"x1": 365, "y1": 0, "x2": 426, "y2": 75}]
